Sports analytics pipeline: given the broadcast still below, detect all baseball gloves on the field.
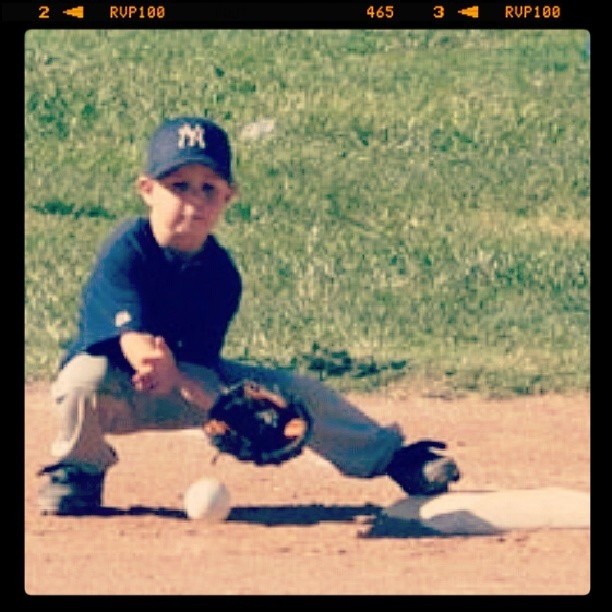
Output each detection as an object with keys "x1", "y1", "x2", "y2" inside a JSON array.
[{"x1": 203, "y1": 381, "x2": 313, "y2": 466}]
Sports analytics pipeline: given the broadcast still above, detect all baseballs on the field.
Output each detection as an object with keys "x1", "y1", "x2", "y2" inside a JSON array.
[{"x1": 183, "y1": 478, "x2": 232, "y2": 525}]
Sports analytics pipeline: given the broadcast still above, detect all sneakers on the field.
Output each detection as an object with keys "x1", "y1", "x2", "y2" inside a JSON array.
[
  {"x1": 387, "y1": 438, "x2": 459, "y2": 493},
  {"x1": 35, "y1": 464, "x2": 104, "y2": 515}
]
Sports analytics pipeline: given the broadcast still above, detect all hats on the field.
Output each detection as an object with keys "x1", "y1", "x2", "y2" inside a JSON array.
[{"x1": 146, "y1": 115, "x2": 231, "y2": 182}]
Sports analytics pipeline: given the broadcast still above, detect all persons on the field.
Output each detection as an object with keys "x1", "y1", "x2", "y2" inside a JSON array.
[{"x1": 36, "y1": 118, "x2": 459, "y2": 515}]
